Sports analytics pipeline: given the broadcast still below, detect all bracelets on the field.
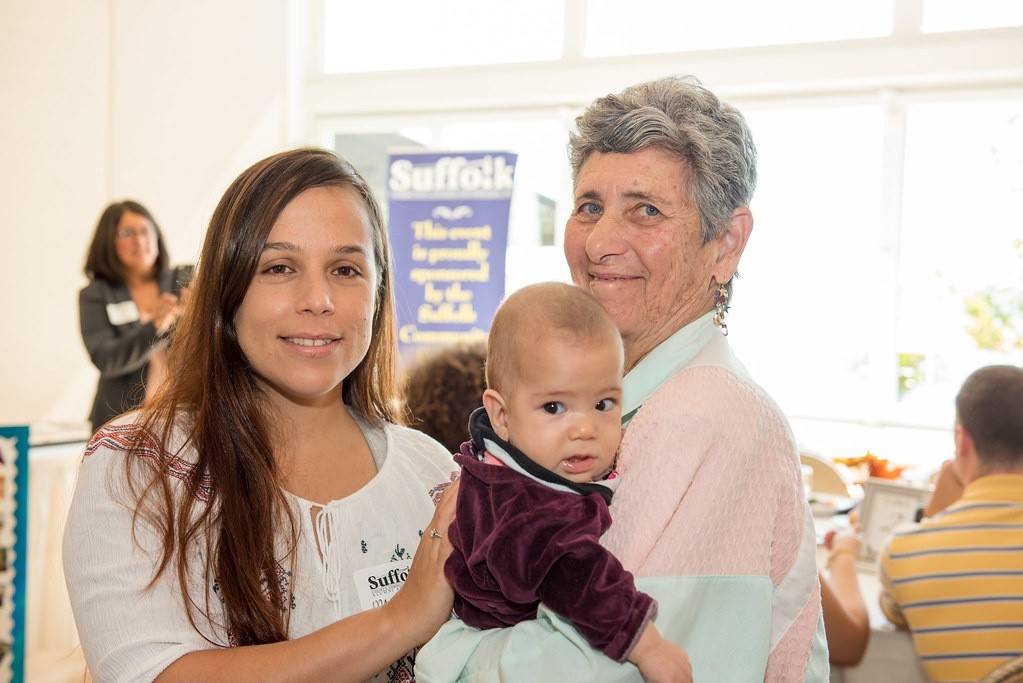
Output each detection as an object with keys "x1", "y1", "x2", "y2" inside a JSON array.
[{"x1": 915, "y1": 506, "x2": 923, "y2": 521}]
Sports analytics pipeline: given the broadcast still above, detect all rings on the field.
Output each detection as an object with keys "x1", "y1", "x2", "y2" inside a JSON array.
[{"x1": 431, "y1": 529, "x2": 442, "y2": 538}]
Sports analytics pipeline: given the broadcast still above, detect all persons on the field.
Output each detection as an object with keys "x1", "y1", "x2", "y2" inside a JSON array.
[
  {"x1": 443, "y1": 281, "x2": 693, "y2": 683},
  {"x1": 414, "y1": 75, "x2": 831, "y2": 683},
  {"x1": 820, "y1": 529, "x2": 869, "y2": 667},
  {"x1": 877, "y1": 364, "x2": 1022, "y2": 683},
  {"x1": 402, "y1": 334, "x2": 489, "y2": 455},
  {"x1": 79, "y1": 201, "x2": 195, "y2": 433},
  {"x1": 62, "y1": 148, "x2": 459, "y2": 683}
]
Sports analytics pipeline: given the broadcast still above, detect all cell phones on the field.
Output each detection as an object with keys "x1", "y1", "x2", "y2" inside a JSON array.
[{"x1": 170, "y1": 264, "x2": 195, "y2": 306}]
